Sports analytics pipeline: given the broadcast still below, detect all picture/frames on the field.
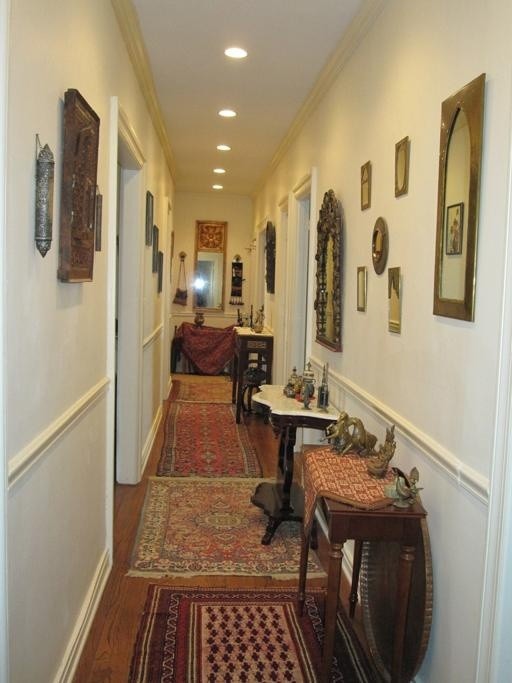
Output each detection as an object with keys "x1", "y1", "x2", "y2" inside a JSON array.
[
  {"x1": 357, "y1": 266, "x2": 366, "y2": 311},
  {"x1": 145, "y1": 192, "x2": 163, "y2": 293},
  {"x1": 446, "y1": 203, "x2": 464, "y2": 255},
  {"x1": 394, "y1": 136, "x2": 409, "y2": 198},
  {"x1": 361, "y1": 160, "x2": 370, "y2": 210},
  {"x1": 57, "y1": 88, "x2": 100, "y2": 283}
]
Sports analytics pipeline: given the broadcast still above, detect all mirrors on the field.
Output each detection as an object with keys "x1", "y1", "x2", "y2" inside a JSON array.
[
  {"x1": 313, "y1": 188, "x2": 343, "y2": 353},
  {"x1": 192, "y1": 220, "x2": 227, "y2": 312},
  {"x1": 433, "y1": 73, "x2": 486, "y2": 321}
]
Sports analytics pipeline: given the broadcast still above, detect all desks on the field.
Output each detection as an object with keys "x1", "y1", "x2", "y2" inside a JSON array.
[
  {"x1": 231, "y1": 327, "x2": 273, "y2": 424},
  {"x1": 298, "y1": 443, "x2": 427, "y2": 683},
  {"x1": 251, "y1": 384, "x2": 340, "y2": 545},
  {"x1": 175, "y1": 323, "x2": 237, "y2": 374}
]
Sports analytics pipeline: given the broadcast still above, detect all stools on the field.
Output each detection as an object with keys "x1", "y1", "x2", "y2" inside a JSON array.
[{"x1": 241, "y1": 368, "x2": 266, "y2": 415}]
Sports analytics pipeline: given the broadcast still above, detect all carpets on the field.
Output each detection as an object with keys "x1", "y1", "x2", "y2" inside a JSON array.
[
  {"x1": 157, "y1": 403, "x2": 263, "y2": 478},
  {"x1": 125, "y1": 477, "x2": 329, "y2": 579},
  {"x1": 169, "y1": 380, "x2": 256, "y2": 403},
  {"x1": 128, "y1": 584, "x2": 377, "y2": 683}
]
